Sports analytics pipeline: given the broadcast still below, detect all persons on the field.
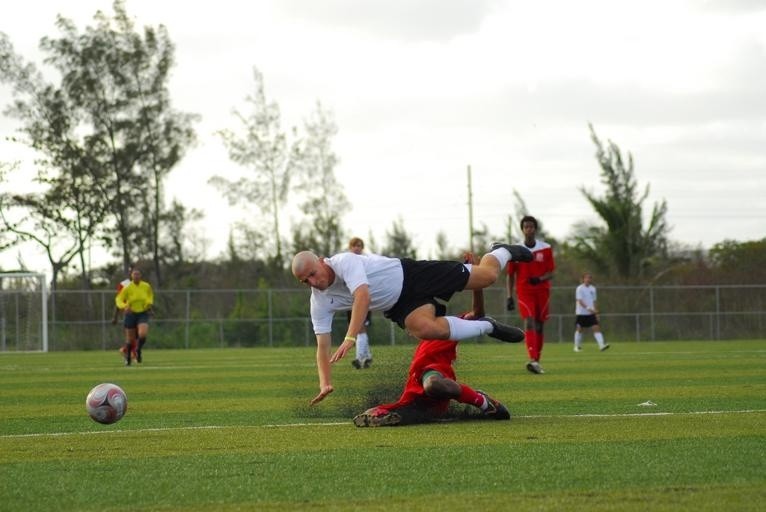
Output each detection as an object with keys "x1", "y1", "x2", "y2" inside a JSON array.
[
  {"x1": 291, "y1": 240, "x2": 534, "y2": 407},
  {"x1": 506, "y1": 215, "x2": 555, "y2": 374},
  {"x1": 573, "y1": 272, "x2": 610, "y2": 352},
  {"x1": 111, "y1": 265, "x2": 139, "y2": 360},
  {"x1": 347, "y1": 237, "x2": 373, "y2": 369},
  {"x1": 352, "y1": 251, "x2": 510, "y2": 428},
  {"x1": 116, "y1": 267, "x2": 155, "y2": 366}
]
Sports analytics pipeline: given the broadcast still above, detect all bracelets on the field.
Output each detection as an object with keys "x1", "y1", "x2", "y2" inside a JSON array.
[
  {"x1": 586, "y1": 306, "x2": 590, "y2": 311},
  {"x1": 345, "y1": 337, "x2": 355, "y2": 342}
]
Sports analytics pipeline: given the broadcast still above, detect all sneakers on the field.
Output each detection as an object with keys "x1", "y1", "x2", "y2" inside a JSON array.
[
  {"x1": 599, "y1": 344, "x2": 611, "y2": 352},
  {"x1": 526, "y1": 359, "x2": 545, "y2": 375},
  {"x1": 125, "y1": 351, "x2": 143, "y2": 367},
  {"x1": 352, "y1": 357, "x2": 373, "y2": 370},
  {"x1": 476, "y1": 315, "x2": 525, "y2": 344},
  {"x1": 490, "y1": 240, "x2": 533, "y2": 265},
  {"x1": 507, "y1": 296, "x2": 515, "y2": 311},
  {"x1": 351, "y1": 409, "x2": 403, "y2": 428},
  {"x1": 573, "y1": 345, "x2": 584, "y2": 352},
  {"x1": 474, "y1": 388, "x2": 510, "y2": 419}
]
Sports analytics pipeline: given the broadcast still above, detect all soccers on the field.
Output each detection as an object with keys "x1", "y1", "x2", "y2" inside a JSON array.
[{"x1": 86, "y1": 382, "x2": 129, "y2": 424}]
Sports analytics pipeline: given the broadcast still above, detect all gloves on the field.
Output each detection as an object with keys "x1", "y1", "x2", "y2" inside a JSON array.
[{"x1": 528, "y1": 277, "x2": 541, "y2": 284}]
